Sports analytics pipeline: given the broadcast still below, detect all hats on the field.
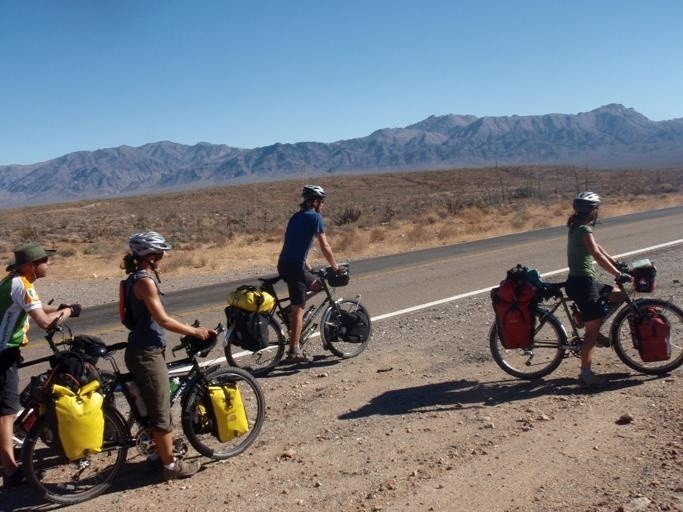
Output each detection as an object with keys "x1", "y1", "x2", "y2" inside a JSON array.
[{"x1": 6, "y1": 244, "x2": 57, "y2": 270}]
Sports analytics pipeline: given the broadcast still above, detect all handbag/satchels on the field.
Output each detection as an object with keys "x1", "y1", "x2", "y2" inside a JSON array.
[
  {"x1": 225, "y1": 285, "x2": 275, "y2": 351},
  {"x1": 491, "y1": 265, "x2": 541, "y2": 348},
  {"x1": 19, "y1": 335, "x2": 107, "y2": 461},
  {"x1": 191, "y1": 377, "x2": 249, "y2": 442},
  {"x1": 328, "y1": 309, "x2": 370, "y2": 343},
  {"x1": 627, "y1": 307, "x2": 671, "y2": 362}
]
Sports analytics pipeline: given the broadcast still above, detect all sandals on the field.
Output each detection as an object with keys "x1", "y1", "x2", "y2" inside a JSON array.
[
  {"x1": 285, "y1": 351, "x2": 315, "y2": 362},
  {"x1": 276, "y1": 310, "x2": 291, "y2": 328}
]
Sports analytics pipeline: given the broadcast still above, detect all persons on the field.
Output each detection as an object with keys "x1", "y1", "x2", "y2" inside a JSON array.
[
  {"x1": 563, "y1": 191, "x2": 634, "y2": 385},
  {"x1": 118, "y1": 231, "x2": 218, "y2": 479},
  {"x1": 277, "y1": 185, "x2": 348, "y2": 362},
  {"x1": 0, "y1": 244, "x2": 81, "y2": 487}
]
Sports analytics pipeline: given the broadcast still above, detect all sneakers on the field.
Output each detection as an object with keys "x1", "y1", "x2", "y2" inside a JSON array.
[
  {"x1": 579, "y1": 375, "x2": 609, "y2": 384},
  {"x1": 597, "y1": 332, "x2": 610, "y2": 348},
  {"x1": 146, "y1": 443, "x2": 202, "y2": 479}
]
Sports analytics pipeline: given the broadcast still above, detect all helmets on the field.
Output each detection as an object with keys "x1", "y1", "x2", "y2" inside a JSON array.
[
  {"x1": 573, "y1": 192, "x2": 601, "y2": 210},
  {"x1": 303, "y1": 185, "x2": 326, "y2": 199},
  {"x1": 129, "y1": 231, "x2": 171, "y2": 256}
]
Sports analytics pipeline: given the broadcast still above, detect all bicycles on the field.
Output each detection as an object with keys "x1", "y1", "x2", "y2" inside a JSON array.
[
  {"x1": 222, "y1": 261, "x2": 372, "y2": 378},
  {"x1": 487, "y1": 258, "x2": 682, "y2": 380},
  {"x1": 20, "y1": 318, "x2": 265, "y2": 506},
  {"x1": 0, "y1": 297, "x2": 136, "y2": 459}
]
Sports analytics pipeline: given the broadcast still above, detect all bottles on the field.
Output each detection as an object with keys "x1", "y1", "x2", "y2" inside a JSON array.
[
  {"x1": 569, "y1": 304, "x2": 583, "y2": 329},
  {"x1": 301, "y1": 305, "x2": 314, "y2": 326},
  {"x1": 166, "y1": 375, "x2": 181, "y2": 400},
  {"x1": 300, "y1": 322, "x2": 317, "y2": 344},
  {"x1": 19, "y1": 410, "x2": 36, "y2": 434},
  {"x1": 600, "y1": 296, "x2": 610, "y2": 312}
]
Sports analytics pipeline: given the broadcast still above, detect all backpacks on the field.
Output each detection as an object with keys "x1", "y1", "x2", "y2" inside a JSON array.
[{"x1": 120, "y1": 276, "x2": 151, "y2": 327}]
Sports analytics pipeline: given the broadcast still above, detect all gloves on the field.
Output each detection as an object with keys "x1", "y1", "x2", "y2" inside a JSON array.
[
  {"x1": 615, "y1": 260, "x2": 630, "y2": 273},
  {"x1": 58, "y1": 303, "x2": 81, "y2": 317},
  {"x1": 338, "y1": 266, "x2": 350, "y2": 282},
  {"x1": 618, "y1": 273, "x2": 632, "y2": 282}
]
